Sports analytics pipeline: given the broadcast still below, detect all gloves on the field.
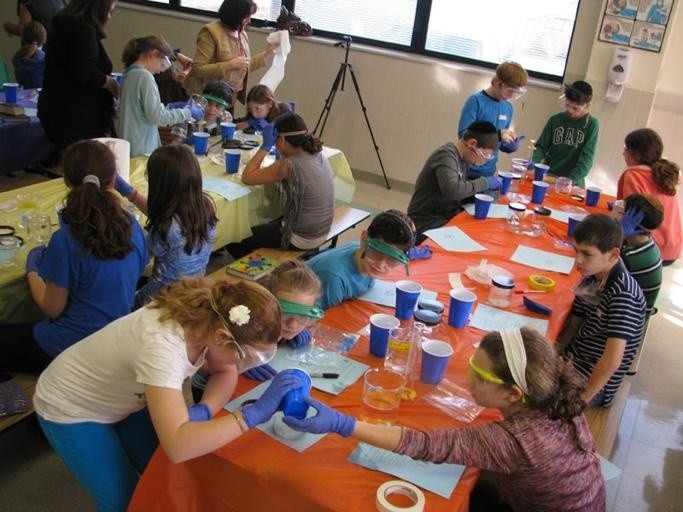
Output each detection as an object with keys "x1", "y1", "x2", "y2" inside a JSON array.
[
  {"x1": 248, "y1": 118, "x2": 278, "y2": 151},
  {"x1": 409, "y1": 245, "x2": 432, "y2": 259},
  {"x1": 242, "y1": 369, "x2": 304, "y2": 429},
  {"x1": 504, "y1": 136, "x2": 524, "y2": 149},
  {"x1": 243, "y1": 363, "x2": 277, "y2": 381},
  {"x1": 26, "y1": 245, "x2": 48, "y2": 277},
  {"x1": 487, "y1": 176, "x2": 502, "y2": 191},
  {"x1": 622, "y1": 207, "x2": 645, "y2": 237},
  {"x1": 115, "y1": 175, "x2": 134, "y2": 197},
  {"x1": 288, "y1": 328, "x2": 311, "y2": 349},
  {"x1": 169, "y1": 98, "x2": 204, "y2": 123},
  {"x1": 283, "y1": 397, "x2": 356, "y2": 438},
  {"x1": 189, "y1": 404, "x2": 211, "y2": 421}
]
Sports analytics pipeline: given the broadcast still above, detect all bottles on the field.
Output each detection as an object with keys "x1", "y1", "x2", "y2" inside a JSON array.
[
  {"x1": 505, "y1": 202, "x2": 553, "y2": 234},
  {"x1": 414, "y1": 299, "x2": 453, "y2": 340},
  {"x1": 490, "y1": 272, "x2": 515, "y2": 308}
]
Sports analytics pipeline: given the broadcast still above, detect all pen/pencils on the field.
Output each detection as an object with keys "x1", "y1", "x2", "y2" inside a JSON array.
[{"x1": 514, "y1": 289, "x2": 546, "y2": 294}]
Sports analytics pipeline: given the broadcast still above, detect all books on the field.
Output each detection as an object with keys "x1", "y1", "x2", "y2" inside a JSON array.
[{"x1": 226, "y1": 252, "x2": 280, "y2": 281}]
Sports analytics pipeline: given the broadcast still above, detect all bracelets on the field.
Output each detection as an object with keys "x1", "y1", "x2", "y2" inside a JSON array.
[{"x1": 129, "y1": 190, "x2": 138, "y2": 202}]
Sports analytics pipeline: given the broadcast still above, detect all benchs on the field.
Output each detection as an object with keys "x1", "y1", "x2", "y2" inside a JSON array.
[
  {"x1": 626, "y1": 317, "x2": 649, "y2": 375},
  {"x1": 0, "y1": 372, "x2": 37, "y2": 431},
  {"x1": 584, "y1": 381, "x2": 631, "y2": 461},
  {"x1": 205, "y1": 205, "x2": 371, "y2": 283}
]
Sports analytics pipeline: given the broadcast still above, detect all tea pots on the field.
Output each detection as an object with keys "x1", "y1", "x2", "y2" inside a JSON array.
[{"x1": 416, "y1": 338, "x2": 454, "y2": 385}]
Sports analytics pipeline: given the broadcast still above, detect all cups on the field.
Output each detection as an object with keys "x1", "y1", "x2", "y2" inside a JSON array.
[
  {"x1": 529, "y1": 163, "x2": 572, "y2": 201},
  {"x1": 3, "y1": 82, "x2": 20, "y2": 103},
  {"x1": 111, "y1": 72, "x2": 123, "y2": 85},
  {"x1": 367, "y1": 311, "x2": 400, "y2": 357},
  {"x1": 474, "y1": 192, "x2": 494, "y2": 220},
  {"x1": 394, "y1": 277, "x2": 422, "y2": 320},
  {"x1": 360, "y1": 368, "x2": 408, "y2": 427},
  {"x1": 447, "y1": 287, "x2": 478, "y2": 328},
  {"x1": 566, "y1": 216, "x2": 585, "y2": 236},
  {"x1": 382, "y1": 325, "x2": 417, "y2": 372},
  {"x1": 272, "y1": 367, "x2": 312, "y2": 442},
  {"x1": 0, "y1": 213, "x2": 51, "y2": 272},
  {"x1": 495, "y1": 158, "x2": 529, "y2": 192},
  {"x1": 193, "y1": 118, "x2": 261, "y2": 175},
  {"x1": 584, "y1": 186, "x2": 601, "y2": 207}
]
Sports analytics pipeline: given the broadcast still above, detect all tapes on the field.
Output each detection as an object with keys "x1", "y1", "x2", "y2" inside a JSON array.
[
  {"x1": 528, "y1": 274, "x2": 555, "y2": 291},
  {"x1": 375, "y1": 480, "x2": 426, "y2": 512}
]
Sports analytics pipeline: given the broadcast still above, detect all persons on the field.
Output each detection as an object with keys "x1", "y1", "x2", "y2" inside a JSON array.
[
  {"x1": 33, "y1": 275, "x2": 304, "y2": 512},
  {"x1": 620, "y1": 194, "x2": 663, "y2": 322},
  {"x1": 4, "y1": 0, "x2": 69, "y2": 38},
  {"x1": 37, "y1": 0, "x2": 120, "y2": 150},
  {"x1": 307, "y1": 208, "x2": 429, "y2": 311},
  {"x1": 191, "y1": 259, "x2": 325, "y2": 404},
  {"x1": 113, "y1": 143, "x2": 219, "y2": 312},
  {"x1": 192, "y1": 0, "x2": 257, "y2": 115},
  {"x1": 225, "y1": 113, "x2": 334, "y2": 260},
  {"x1": 12, "y1": 20, "x2": 47, "y2": 89},
  {"x1": 183, "y1": 81, "x2": 235, "y2": 144},
  {"x1": 25, "y1": 139, "x2": 149, "y2": 371},
  {"x1": 153, "y1": 50, "x2": 194, "y2": 107},
  {"x1": 117, "y1": 34, "x2": 205, "y2": 157},
  {"x1": 234, "y1": 85, "x2": 290, "y2": 134},
  {"x1": 458, "y1": 63, "x2": 528, "y2": 213},
  {"x1": 534, "y1": 81, "x2": 599, "y2": 189},
  {"x1": 409, "y1": 120, "x2": 503, "y2": 246},
  {"x1": 555, "y1": 213, "x2": 646, "y2": 407},
  {"x1": 283, "y1": 327, "x2": 607, "y2": 512},
  {"x1": 618, "y1": 128, "x2": 683, "y2": 266}
]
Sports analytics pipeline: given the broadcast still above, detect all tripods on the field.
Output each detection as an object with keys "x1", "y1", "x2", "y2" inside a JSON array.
[{"x1": 313, "y1": 34, "x2": 392, "y2": 190}]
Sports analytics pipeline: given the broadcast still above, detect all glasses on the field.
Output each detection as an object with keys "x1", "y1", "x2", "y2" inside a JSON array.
[
  {"x1": 466, "y1": 355, "x2": 526, "y2": 404},
  {"x1": 501, "y1": 84, "x2": 527, "y2": 100},
  {"x1": 278, "y1": 300, "x2": 324, "y2": 325},
  {"x1": 559, "y1": 94, "x2": 586, "y2": 113},
  {"x1": 160, "y1": 56, "x2": 172, "y2": 72},
  {"x1": 247, "y1": 104, "x2": 267, "y2": 112},
  {"x1": 466, "y1": 144, "x2": 495, "y2": 166},
  {"x1": 366, "y1": 235, "x2": 409, "y2": 269},
  {"x1": 202, "y1": 94, "x2": 229, "y2": 110},
  {"x1": 611, "y1": 200, "x2": 625, "y2": 223},
  {"x1": 218, "y1": 340, "x2": 277, "y2": 374},
  {"x1": 570, "y1": 272, "x2": 609, "y2": 305}
]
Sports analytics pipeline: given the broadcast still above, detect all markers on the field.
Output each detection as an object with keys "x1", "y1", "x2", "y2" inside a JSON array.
[{"x1": 309, "y1": 372, "x2": 339, "y2": 379}]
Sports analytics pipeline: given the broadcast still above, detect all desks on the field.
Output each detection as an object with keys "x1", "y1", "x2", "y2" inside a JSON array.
[
  {"x1": 128, "y1": 174, "x2": 618, "y2": 511},
  {"x1": 0, "y1": 89, "x2": 54, "y2": 177},
  {"x1": 0, "y1": 135, "x2": 357, "y2": 325}
]
